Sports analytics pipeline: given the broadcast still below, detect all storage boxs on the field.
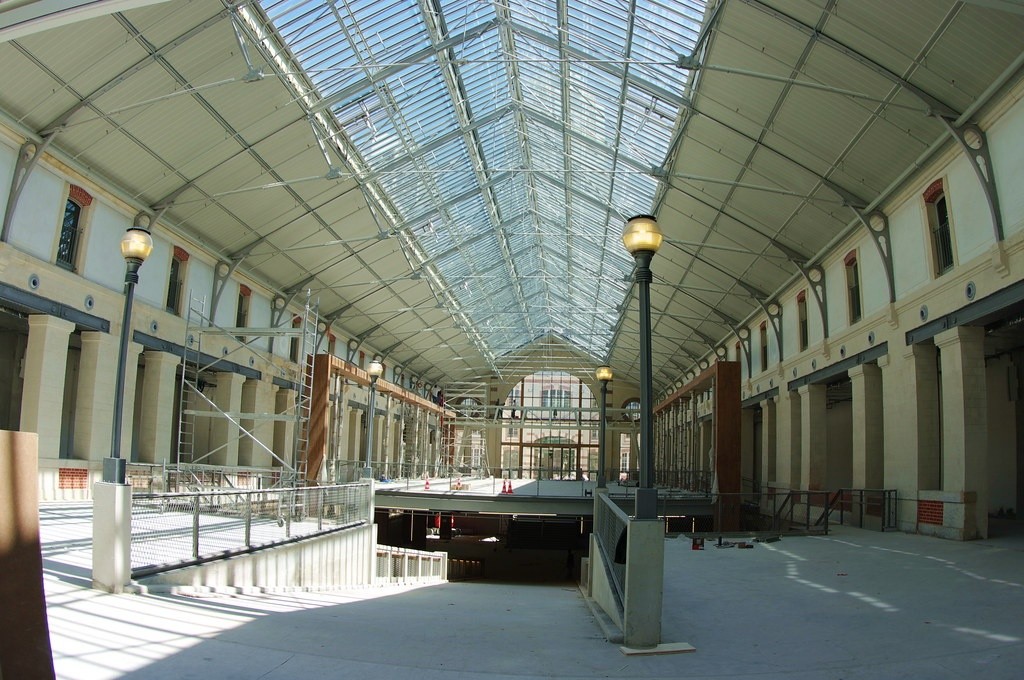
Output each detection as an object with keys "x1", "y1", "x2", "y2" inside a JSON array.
[
  {"x1": 458, "y1": 485, "x2": 465, "y2": 490},
  {"x1": 450, "y1": 485, "x2": 458, "y2": 490},
  {"x1": 692, "y1": 537, "x2": 704, "y2": 550}
]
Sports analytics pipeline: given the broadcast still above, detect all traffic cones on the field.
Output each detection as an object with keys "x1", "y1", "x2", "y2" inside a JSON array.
[
  {"x1": 425, "y1": 477, "x2": 430, "y2": 490},
  {"x1": 457, "y1": 476, "x2": 462, "y2": 488},
  {"x1": 506, "y1": 480, "x2": 514, "y2": 495},
  {"x1": 498, "y1": 480, "x2": 507, "y2": 495}
]
